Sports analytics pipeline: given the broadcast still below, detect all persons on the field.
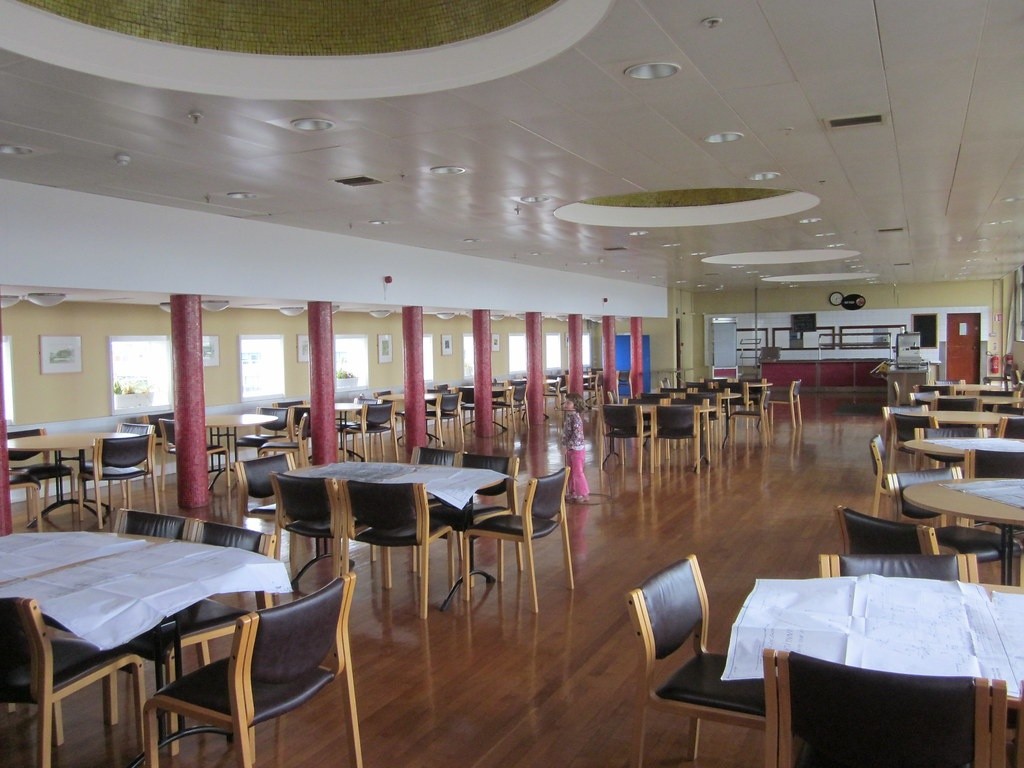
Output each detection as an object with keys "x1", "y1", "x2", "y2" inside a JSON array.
[{"x1": 559, "y1": 393, "x2": 591, "y2": 503}]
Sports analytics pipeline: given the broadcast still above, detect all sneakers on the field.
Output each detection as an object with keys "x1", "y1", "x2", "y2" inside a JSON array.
[
  {"x1": 574, "y1": 495, "x2": 590, "y2": 503},
  {"x1": 565, "y1": 494, "x2": 576, "y2": 500}
]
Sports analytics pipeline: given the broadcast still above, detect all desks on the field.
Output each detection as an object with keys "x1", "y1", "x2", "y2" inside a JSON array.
[
  {"x1": 748, "y1": 382, "x2": 774, "y2": 429},
  {"x1": 561, "y1": 375, "x2": 596, "y2": 410},
  {"x1": 947, "y1": 385, "x2": 1011, "y2": 397},
  {"x1": 906, "y1": 438, "x2": 1023, "y2": 467},
  {"x1": 720, "y1": 573, "x2": 1023, "y2": 709},
  {"x1": 902, "y1": 477, "x2": 1023, "y2": 584},
  {"x1": 513, "y1": 379, "x2": 557, "y2": 419},
  {"x1": 937, "y1": 395, "x2": 1024, "y2": 412},
  {"x1": 601, "y1": 401, "x2": 717, "y2": 471},
  {"x1": 905, "y1": 412, "x2": 1018, "y2": 438},
  {"x1": 721, "y1": 392, "x2": 742, "y2": 446},
  {"x1": 203, "y1": 415, "x2": 278, "y2": 490},
  {"x1": 5, "y1": 433, "x2": 140, "y2": 527},
  {"x1": 458, "y1": 386, "x2": 508, "y2": 431},
  {"x1": 379, "y1": 393, "x2": 447, "y2": 445},
  {"x1": 288, "y1": 403, "x2": 366, "y2": 462},
  {"x1": 0, "y1": 531, "x2": 293, "y2": 768},
  {"x1": 287, "y1": 461, "x2": 511, "y2": 612}
]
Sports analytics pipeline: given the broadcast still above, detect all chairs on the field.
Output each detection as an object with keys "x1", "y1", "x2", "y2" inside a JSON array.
[{"x1": 0, "y1": 368, "x2": 1024, "y2": 768}]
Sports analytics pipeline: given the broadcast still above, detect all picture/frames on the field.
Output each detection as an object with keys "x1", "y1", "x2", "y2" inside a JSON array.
[{"x1": 39, "y1": 333, "x2": 502, "y2": 374}]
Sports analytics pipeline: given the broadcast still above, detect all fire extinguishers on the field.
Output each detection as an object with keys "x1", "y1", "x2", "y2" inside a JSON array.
[
  {"x1": 1007, "y1": 352, "x2": 1013, "y2": 365},
  {"x1": 992, "y1": 354, "x2": 999, "y2": 373}
]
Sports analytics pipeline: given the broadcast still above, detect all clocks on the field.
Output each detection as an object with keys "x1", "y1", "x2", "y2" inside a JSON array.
[{"x1": 829, "y1": 291, "x2": 844, "y2": 306}]
